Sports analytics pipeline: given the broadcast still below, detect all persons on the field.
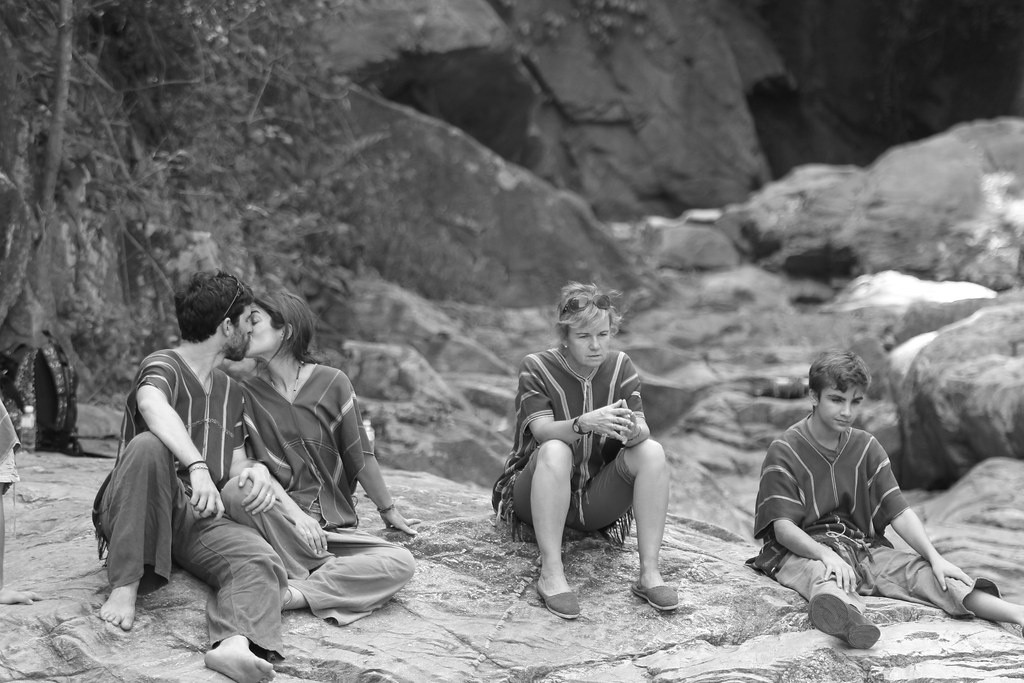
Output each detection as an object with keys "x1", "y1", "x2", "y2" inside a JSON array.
[
  {"x1": 240, "y1": 291, "x2": 421, "y2": 628},
  {"x1": 0, "y1": 398, "x2": 22, "y2": 589},
  {"x1": 91, "y1": 268, "x2": 288, "y2": 682},
  {"x1": 493, "y1": 282, "x2": 678, "y2": 619},
  {"x1": 743, "y1": 349, "x2": 1024, "y2": 649}
]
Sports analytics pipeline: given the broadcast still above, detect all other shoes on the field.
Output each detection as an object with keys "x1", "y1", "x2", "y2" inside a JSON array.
[
  {"x1": 631, "y1": 579, "x2": 680, "y2": 610},
  {"x1": 536, "y1": 581, "x2": 580, "y2": 618},
  {"x1": 807, "y1": 593, "x2": 881, "y2": 650}
]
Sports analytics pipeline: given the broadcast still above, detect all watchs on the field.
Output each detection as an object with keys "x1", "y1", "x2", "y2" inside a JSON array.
[{"x1": 573, "y1": 416, "x2": 593, "y2": 439}]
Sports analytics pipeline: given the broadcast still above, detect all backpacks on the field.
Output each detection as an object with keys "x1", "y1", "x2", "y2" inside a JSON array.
[{"x1": 0, "y1": 330, "x2": 84, "y2": 458}]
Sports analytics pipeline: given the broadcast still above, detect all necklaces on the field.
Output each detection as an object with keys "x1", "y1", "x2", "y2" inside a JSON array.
[{"x1": 267, "y1": 361, "x2": 304, "y2": 404}]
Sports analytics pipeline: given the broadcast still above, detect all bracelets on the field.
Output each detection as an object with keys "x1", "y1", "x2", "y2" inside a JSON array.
[
  {"x1": 376, "y1": 503, "x2": 394, "y2": 513},
  {"x1": 187, "y1": 460, "x2": 209, "y2": 474}
]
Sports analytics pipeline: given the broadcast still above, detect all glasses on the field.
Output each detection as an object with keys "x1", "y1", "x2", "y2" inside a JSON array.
[
  {"x1": 559, "y1": 295, "x2": 611, "y2": 319},
  {"x1": 217, "y1": 272, "x2": 244, "y2": 325}
]
